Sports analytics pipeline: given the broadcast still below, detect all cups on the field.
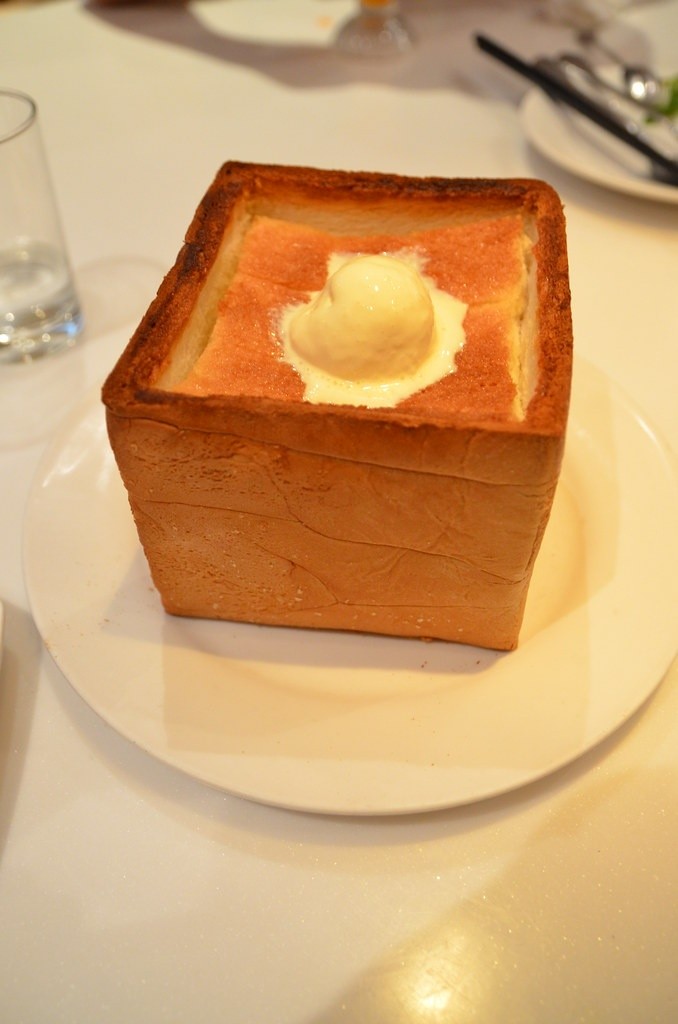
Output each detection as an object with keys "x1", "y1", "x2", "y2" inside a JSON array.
[{"x1": 2, "y1": 88, "x2": 83, "y2": 370}]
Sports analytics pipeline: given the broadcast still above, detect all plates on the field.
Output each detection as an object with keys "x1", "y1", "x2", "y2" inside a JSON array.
[
  {"x1": 24, "y1": 323, "x2": 675, "y2": 821},
  {"x1": 519, "y1": 14, "x2": 678, "y2": 206}
]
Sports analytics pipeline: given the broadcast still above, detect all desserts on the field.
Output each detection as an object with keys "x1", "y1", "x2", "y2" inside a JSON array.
[{"x1": 101, "y1": 159, "x2": 575, "y2": 651}]
